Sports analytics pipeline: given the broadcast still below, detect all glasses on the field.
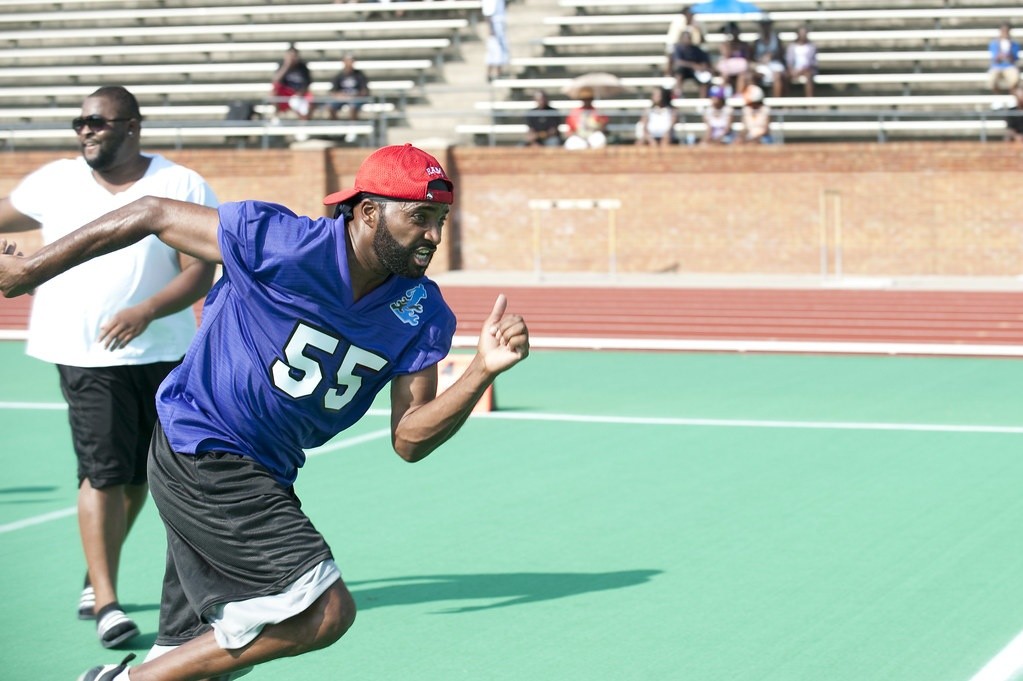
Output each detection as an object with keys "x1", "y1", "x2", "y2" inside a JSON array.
[{"x1": 70, "y1": 115, "x2": 132, "y2": 132}]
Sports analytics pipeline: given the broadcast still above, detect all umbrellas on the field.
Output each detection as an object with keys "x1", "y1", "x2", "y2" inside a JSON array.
[{"x1": 688, "y1": 0, "x2": 766, "y2": 13}]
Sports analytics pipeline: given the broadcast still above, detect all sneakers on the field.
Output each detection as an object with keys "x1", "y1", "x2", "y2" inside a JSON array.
[{"x1": 79, "y1": 653, "x2": 135, "y2": 679}]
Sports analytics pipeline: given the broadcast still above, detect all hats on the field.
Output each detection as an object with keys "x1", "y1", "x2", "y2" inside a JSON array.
[{"x1": 320, "y1": 142, "x2": 456, "y2": 206}]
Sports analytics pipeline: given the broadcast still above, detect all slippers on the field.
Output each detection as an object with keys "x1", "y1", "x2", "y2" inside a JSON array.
[
  {"x1": 80, "y1": 578, "x2": 97, "y2": 619},
  {"x1": 96, "y1": 604, "x2": 138, "y2": 652}
]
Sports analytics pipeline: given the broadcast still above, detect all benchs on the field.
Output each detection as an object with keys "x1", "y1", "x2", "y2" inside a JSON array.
[{"x1": 0, "y1": 3, "x2": 1023, "y2": 142}]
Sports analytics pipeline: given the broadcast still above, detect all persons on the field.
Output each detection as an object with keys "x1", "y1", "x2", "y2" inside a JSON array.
[
  {"x1": 480, "y1": 0, "x2": 513, "y2": 82},
  {"x1": 323, "y1": 52, "x2": 369, "y2": 121},
  {"x1": 698, "y1": 91, "x2": 733, "y2": 145},
  {"x1": 718, "y1": 26, "x2": 751, "y2": 95},
  {"x1": 328, "y1": 0, "x2": 470, "y2": 26},
  {"x1": 717, "y1": 27, "x2": 726, "y2": 34},
  {"x1": 0, "y1": 145, "x2": 530, "y2": 680},
  {"x1": 519, "y1": 89, "x2": 562, "y2": 147},
  {"x1": 978, "y1": 20, "x2": 1020, "y2": 95},
  {"x1": 701, "y1": 34, "x2": 706, "y2": 43},
  {"x1": 753, "y1": 51, "x2": 785, "y2": 87},
  {"x1": 665, "y1": 6, "x2": 701, "y2": 51},
  {"x1": 785, "y1": 24, "x2": 819, "y2": 112},
  {"x1": 633, "y1": 85, "x2": 677, "y2": 145},
  {"x1": 271, "y1": 39, "x2": 317, "y2": 120},
  {"x1": 727, "y1": 22, "x2": 740, "y2": 34},
  {"x1": 0, "y1": 81, "x2": 225, "y2": 649},
  {"x1": 667, "y1": 31, "x2": 714, "y2": 98},
  {"x1": 745, "y1": 19, "x2": 786, "y2": 99},
  {"x1": 562, "y1": 87, "x2": 610, "y2": 149},
  {"x1": 735, "y1": 84, "x2": 774, "y2": 146},
  {"x1": 1002, "y1": 78, "x2": 1023, "y2": 141}
]
